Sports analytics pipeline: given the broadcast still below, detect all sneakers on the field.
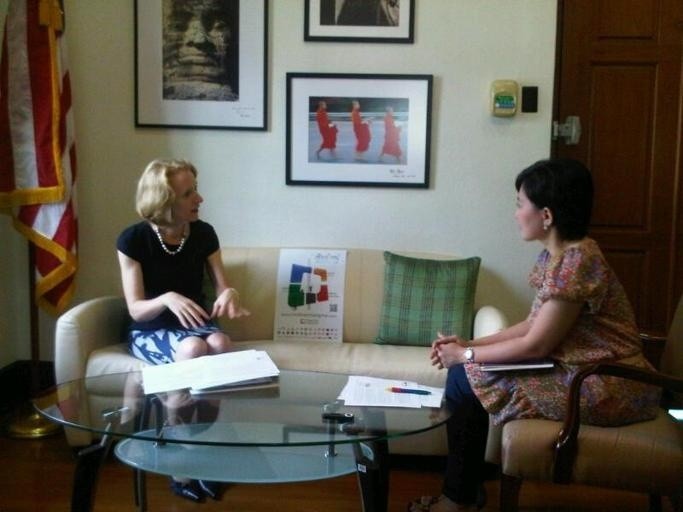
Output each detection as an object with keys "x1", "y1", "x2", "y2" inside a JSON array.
[{"x1": 406, "y1": 495, "x2": 466, "y2": 511}]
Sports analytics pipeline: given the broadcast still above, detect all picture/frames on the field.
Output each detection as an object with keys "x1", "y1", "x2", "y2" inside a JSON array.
[
  {"x1": 304, "y1": 0, "x2": 416, "y2": 44},
  {"x1": 134, "y1": 0, "x2": 269, "y2": 132},
  {"x1": 286, "y1": 71, "x2": 433, "y2": 189}
]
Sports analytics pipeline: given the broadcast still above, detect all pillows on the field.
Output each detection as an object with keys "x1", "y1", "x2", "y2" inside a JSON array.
[{"x1": 375, "y1": 251, "x2": 481, "y2": 347}]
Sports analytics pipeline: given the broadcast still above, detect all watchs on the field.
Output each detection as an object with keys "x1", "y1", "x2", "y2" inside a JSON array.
[{"x1": 464, "y1": 344, "x2": 476, "y2": 365}]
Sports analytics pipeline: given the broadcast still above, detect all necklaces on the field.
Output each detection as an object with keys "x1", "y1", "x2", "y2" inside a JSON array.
[{"x1": 149, "y1": 218, "x2": 188, "y2": 255}]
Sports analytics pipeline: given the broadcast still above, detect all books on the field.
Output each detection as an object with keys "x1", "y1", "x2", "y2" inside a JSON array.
[
  {"x1": 187, "y1": 349, "x2": 280, "y2": 396},
  {"x1": 478, "y1": 360, "x2": 555, "y2": 374}
]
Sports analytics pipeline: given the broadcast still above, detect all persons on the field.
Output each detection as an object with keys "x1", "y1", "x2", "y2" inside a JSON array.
[
  {"x1": 352, "y1": 99, "x2": 372, "y2": 161},
  {"x1": 115, "y1": 158, "x2": 251, "y2": 503},
  {"x1": 162, "y1": 1, "x2": 239, "y2": 101},
  {"x1": 314, "y1": 100, "x2": 340, "y2": 160},
  {"x1": 378, "y1": 106, "x2": 405, "y2": 164},
  {"x1": 408, "y1": 159, "x2": 664, "y2": 511}
]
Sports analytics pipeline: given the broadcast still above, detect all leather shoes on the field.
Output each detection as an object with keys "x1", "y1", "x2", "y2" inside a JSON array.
[
  {"x1": 197, "y1": 479, "x2": 221, "y2": 501},
  {"x1": 170, "y1": 481, "x2": 203, "y2": 503}
]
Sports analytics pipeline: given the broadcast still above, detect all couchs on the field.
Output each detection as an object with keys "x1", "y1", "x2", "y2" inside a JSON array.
[{"x1": 54, "y1": 246, "x2": 507, "y2": 465}]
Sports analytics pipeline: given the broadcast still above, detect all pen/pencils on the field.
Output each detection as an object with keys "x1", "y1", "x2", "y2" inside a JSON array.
[{"x1": 384, "y1": 386, "x2": 430, "y2": 396}]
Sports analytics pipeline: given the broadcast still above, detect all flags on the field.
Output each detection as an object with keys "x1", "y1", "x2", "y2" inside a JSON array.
[{"x1": 1, "y1": 0, "x2": 81, "y2": 322}]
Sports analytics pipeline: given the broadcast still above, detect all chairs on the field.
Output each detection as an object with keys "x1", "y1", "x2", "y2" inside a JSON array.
[{"x1": 499, "y1": 299, "x2": 683, "y2": 512}]
